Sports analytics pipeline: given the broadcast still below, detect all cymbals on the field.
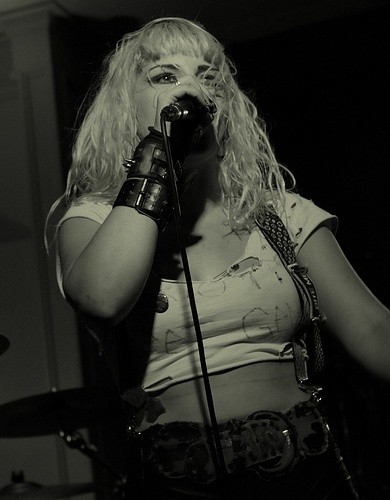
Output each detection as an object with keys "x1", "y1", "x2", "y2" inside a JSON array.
[
  {"x1": 0, "y1": 383, "x2": 122, "y2": 438},
  {"x1": 0, "y1": 335, "x2": 11, "y2": 356},
  {"x1": 0, "y1": 479, "x2": 99, "y2": 500}
]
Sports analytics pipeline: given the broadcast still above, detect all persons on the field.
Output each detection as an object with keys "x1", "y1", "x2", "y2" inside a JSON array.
[{"x1": 42, "y1": 13, "x2": 390, "y2": 500}]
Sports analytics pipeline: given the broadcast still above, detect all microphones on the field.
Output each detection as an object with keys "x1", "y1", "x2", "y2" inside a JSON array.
[{"x1": 161, "y1": 98, "x2": 210, "y2": 122}]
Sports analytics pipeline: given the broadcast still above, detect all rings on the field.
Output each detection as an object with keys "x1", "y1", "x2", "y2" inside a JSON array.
[{"x1": 174, "y1": 79, "x2": 181, "y2": 87}]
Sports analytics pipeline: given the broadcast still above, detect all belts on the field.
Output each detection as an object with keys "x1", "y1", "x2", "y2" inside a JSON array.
[{"x1": 128, "y1": 402, "x2": 331, "y2": 486}]
